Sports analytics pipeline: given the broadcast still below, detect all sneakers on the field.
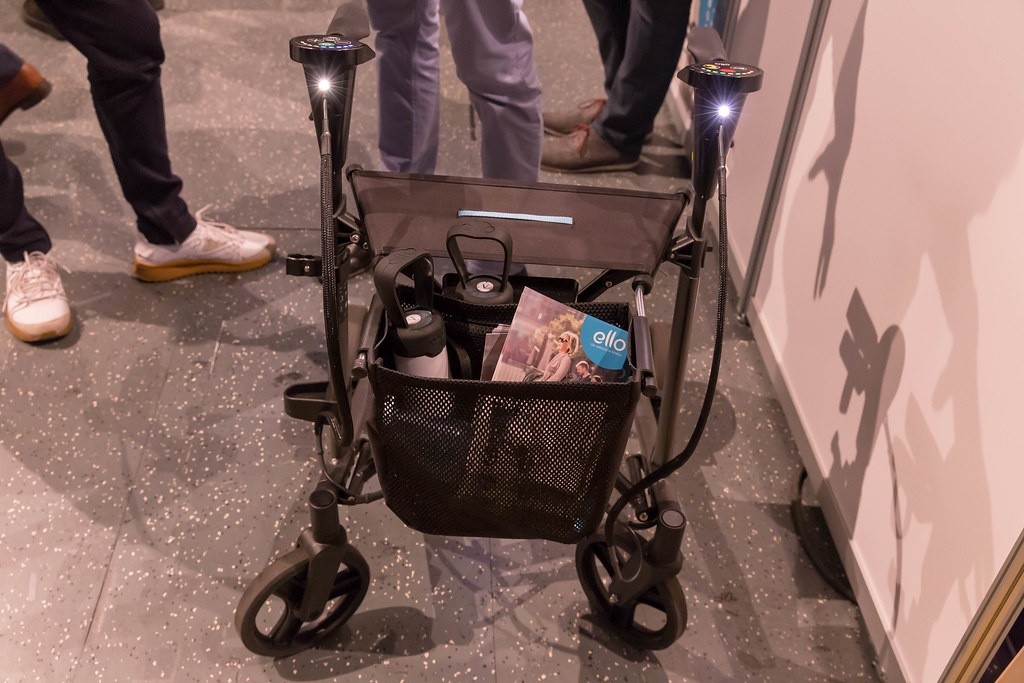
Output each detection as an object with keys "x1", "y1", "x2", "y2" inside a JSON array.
[
  {"x1": 134, "y1": 202, "x2": 276, "y2": 281},
  {"x1": 1, "y1": 249, "x2": 72, "y2": 341},
  {"x1": 540, "y1": 122, "x2": 640, "y2": 172},
  {"x1": 541, "y1": 97, "x2": 654, "y2": 142}
]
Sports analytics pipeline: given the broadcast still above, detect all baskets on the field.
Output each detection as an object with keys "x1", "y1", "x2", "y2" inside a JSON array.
[{"x1": 368, "y1": 283, "x2": 639, "y2": 544}]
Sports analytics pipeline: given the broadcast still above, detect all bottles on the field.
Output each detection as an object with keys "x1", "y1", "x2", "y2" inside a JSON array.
[{"x1": 392, "y1": 306, "x2": 457, "y2": 419}]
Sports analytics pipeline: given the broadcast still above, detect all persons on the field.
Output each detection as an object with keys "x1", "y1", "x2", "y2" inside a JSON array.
[
  {"x1": 319, "y1": 0, "x2": 545, "y2": 282},
  {"x1": 533, "y1": 0, "x2": 693, "y2": 171},
  {"x1": 0, "y1": 0, "x2": 276, "y2": 344},
  {"x1": 522, "y1": 331, "x2": 602, "y2": 383}
]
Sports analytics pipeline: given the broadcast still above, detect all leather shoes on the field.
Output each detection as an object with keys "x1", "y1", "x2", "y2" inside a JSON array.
[{"x1": 0, "y1": 61, "x2": 52, "y2": 125}]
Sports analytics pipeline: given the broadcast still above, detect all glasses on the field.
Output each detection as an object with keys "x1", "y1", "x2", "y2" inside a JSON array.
[{"x1": 558, "y1": 337, "x2": 567, "y2": 342}]
